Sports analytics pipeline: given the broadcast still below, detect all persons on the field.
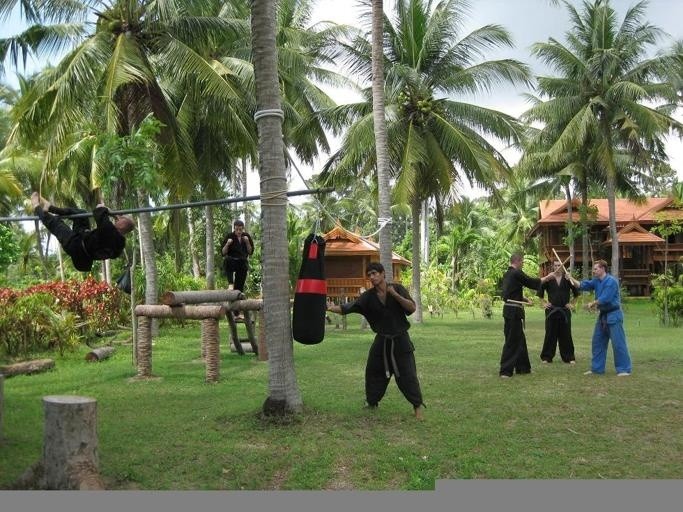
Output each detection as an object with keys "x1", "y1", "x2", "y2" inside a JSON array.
[
  {"x1": 326, "y1": 262, "x2": 426, "y2": 422},
  {"x1": 219, "y1": 220, "x2": 254, "y2": 321},
  {"x1": 565, "y1": 260, "x2": 633, "y2": 377},
  {"x1": 535, "y1": 259, "x2": 580, "y2": 368},
  {"x1": 31, "y1": 190, "x2": 135, "y2": 272},
  {"x1": 497, "y1": 251, "x2": 555, "y2": 381}
]
